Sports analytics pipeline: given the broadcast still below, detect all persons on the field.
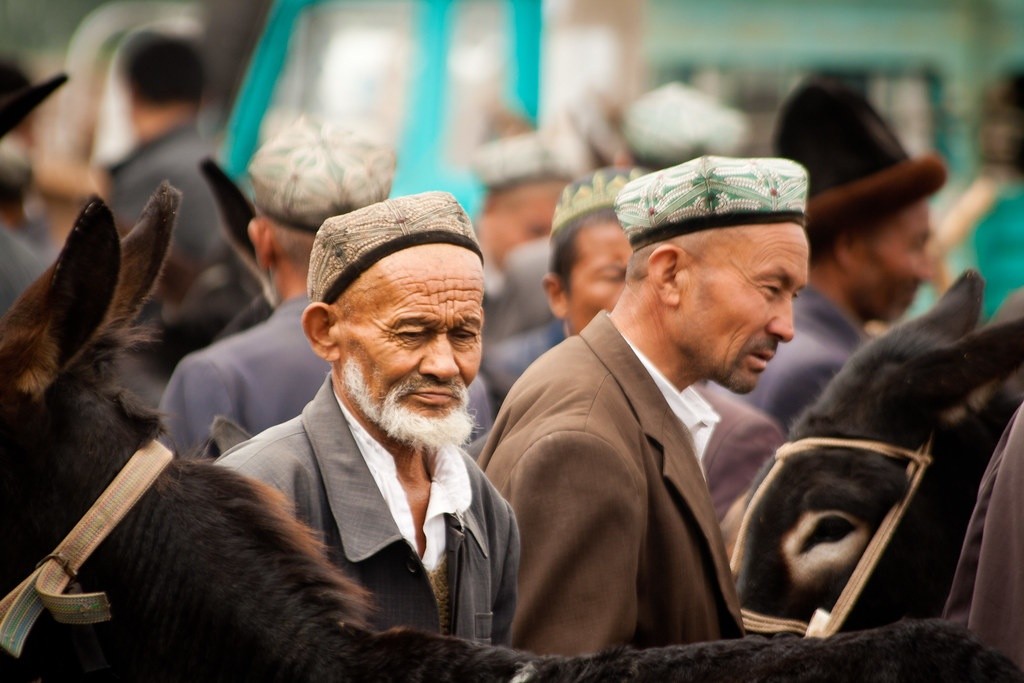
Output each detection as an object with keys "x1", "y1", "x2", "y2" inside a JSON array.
[
  {"x1": 478, "y1": 73, "x2": 748, "y2": 341},
  {"x1": 152, "y1": 114, "x2": 494, "y2": 461},
  {"x1": 0, "y1": 137, "x2": 64, "y2": 322},
  {"x1": 91, "y1": 25, "x2": 228, "y2": 271},
  {"x1": 211, "y1": 189, "x2": 519, "y2": 653},
  {"x1": 730, "y1": 76, "x2": 947, "y2": 432},
  {"x1": 471, "y1": 157, "x2": 810, "y2": 658},
  {"x1": 482, "y1": 170, "x2": 781, "y2": 523}
]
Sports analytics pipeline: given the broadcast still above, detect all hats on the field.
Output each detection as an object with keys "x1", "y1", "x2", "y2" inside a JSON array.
[
  {"x1": 613, "y1": 155, "x2": 807, "y2": 252},
  {"x1": 771, "y1": 75, "x2": 946, "y2": 243},
  {"x1": 247, "y1": 116, "x2": 397, "y2": 233},
  {"x1": 550, "y1": 167, "x2": 645, "y2": 238},
  {"x1": 306, "y1": 190, "x2": 485, "y2": 301}
]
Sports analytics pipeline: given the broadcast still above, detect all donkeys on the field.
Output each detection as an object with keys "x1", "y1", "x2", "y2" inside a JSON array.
[
  {"x1": 137, "y1": 154, "x2": 281, "y2": 378},
  {"x1": 0, "y1": 171, "x2": 1024, "y2": 683},
  {"x1": 735, "y1": 270, "x2": 1023, "y2": 639}
]
